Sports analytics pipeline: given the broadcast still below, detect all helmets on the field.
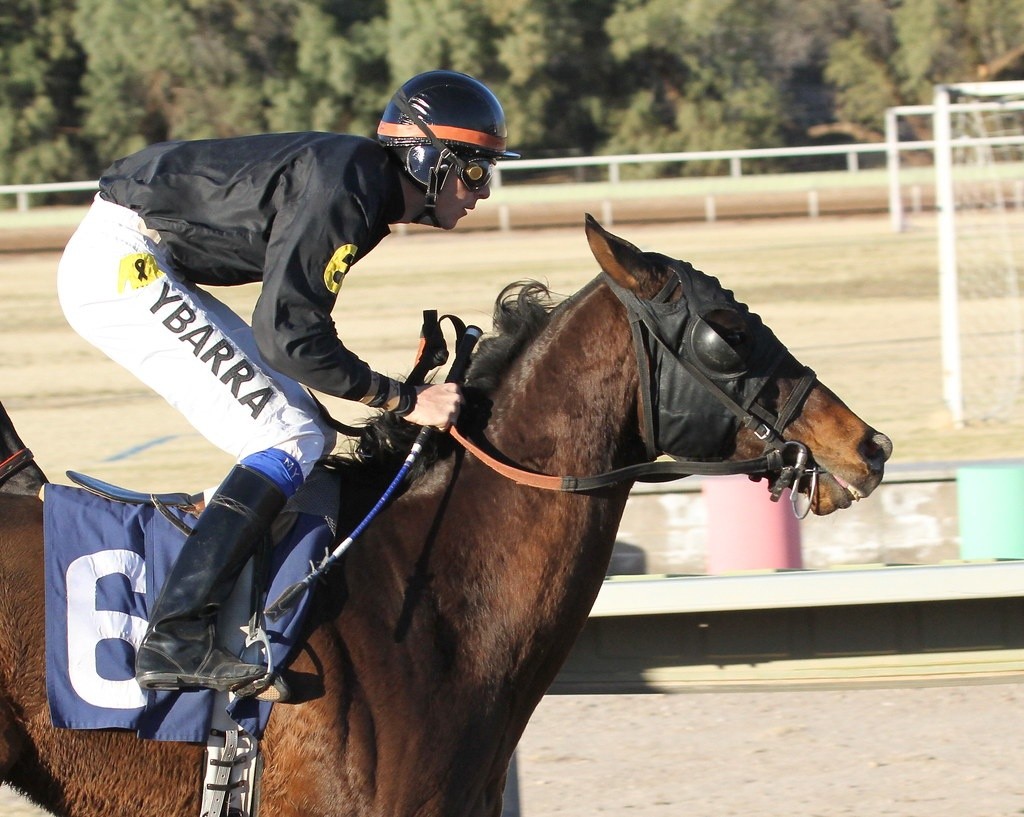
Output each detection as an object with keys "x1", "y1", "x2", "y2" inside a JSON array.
[{"x1": 378, "y1": 71, "x2": 522, "y2": 159}]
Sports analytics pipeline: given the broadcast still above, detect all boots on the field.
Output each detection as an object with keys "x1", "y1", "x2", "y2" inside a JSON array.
[{"x1": 135, "y1": 463, "x2": 268, "y2": 691}]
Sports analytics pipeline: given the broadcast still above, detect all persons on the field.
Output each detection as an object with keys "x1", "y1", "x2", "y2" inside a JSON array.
[{"x1": 56, "y1": 72, "x2": 521, "y2": 703}]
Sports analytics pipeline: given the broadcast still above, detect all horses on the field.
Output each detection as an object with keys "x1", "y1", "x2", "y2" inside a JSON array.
[{"x1": 0, "y1": 211, "x2": 896, "y2": 817}]
